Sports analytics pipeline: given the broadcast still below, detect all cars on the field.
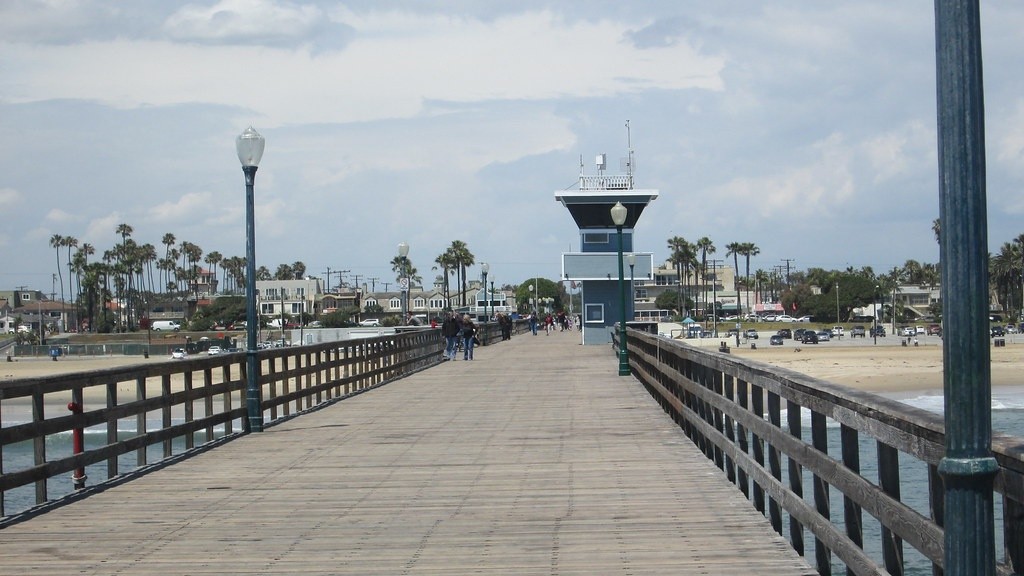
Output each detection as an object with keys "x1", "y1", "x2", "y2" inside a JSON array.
[
  {"x1": 225, "y1": 339, "x2": 305, "y2": 353},
  {"x1": 702, "y1": 313, "x2": 816, "y2": 323},
  {"x1": 8, "y1": 326, "x2": 29, "y2": 334},
  {"x1": 897, "y1": 323, "x2": 941, "y2": 336},
  {"x1": 267, "y1": 319, "x2": 326, "y2": 328},
  {"x1": 769, "y1": 328, "x2": 831, "y2": 345},
  {"x1": 831, "y1": 326, "x2": 844, "y2": 336},
  {"x1": 744, "y1": 329, "x2": 758, "y2": 339},
  {"x1": 989, "y1": 314, "x2": 1024, "y2": 336},
  {"x1": 207, "y1": 346, "x2": 222, "y2": 355},
  {"x1": 729, "y1": 328, "x2": 740, "y2": 336}
]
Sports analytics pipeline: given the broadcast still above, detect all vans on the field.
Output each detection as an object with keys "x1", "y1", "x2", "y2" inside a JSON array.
[
  {"x1": 687, "y1": 324, "x2": 703, "y2": 337},
  {"x1": 152, "y1": 320, "x2": 181, "y2": 331},
  {"x1": 358, "y1": 319, "x2": 379, "y2": 327}
]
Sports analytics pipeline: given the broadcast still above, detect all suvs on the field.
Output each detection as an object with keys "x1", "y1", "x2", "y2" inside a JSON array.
[
  {"x1": 850, "y1": 326, "x2": 865, "y2": 337},
  {"x1": 870, "y1": 326, "x2": 886, "y2": 337}
]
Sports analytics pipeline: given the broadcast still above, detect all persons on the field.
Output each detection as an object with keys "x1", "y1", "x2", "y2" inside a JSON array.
[
  {"x1": 496, "y1": 312, "x2": 512, "y2": 341},
  {"x1": 545, "y1": 311, "x2": 582, "y2": 335},
  {"x1": 527, "y1": 311, "x2": 538, "y2": 335},
  {"x1": 442, "y1": 312, "x2": 478, "y2": 360},
  {"x1": 406, "y1": 312, "x2": 422, "y2": 326}
]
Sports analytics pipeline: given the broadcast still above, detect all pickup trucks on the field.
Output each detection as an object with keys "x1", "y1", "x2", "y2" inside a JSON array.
[{"x1": 171, "y1": 348, "x2": 188, "y2": 359}]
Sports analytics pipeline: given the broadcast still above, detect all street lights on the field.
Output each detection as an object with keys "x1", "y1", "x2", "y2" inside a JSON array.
[
  {"x1": 626, "y1": 253, "x2": 636, "y2": 320},
  {"x1": 396, "y1": 241, "x2": 411, "y2": 325},
  {"x1": 528, "y1": 285, "x2": 553, "y2": 313},
  {"x1": 482, "y1": 263, "x2": 490, "y2": 346},
  {"x1": 237, "y1": 124, "x2": 266, "y2": 433},
  {"x1": 489, "y1": 272, "x2": 495, "y2": 318},
  {"x1": 873, "y1": 285, "x2": 880, "y2": 345},
  {"x1": 611, "y1": 201, "x2": 631, "y2": 376}
]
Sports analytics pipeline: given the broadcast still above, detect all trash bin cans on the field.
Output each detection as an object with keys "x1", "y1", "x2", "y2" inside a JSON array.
[
  {"x1": 914, "y1": 340, "x2": 918, "y2": 346},
  {"x1": 751, "y1": 343, "x2": 755, "y2": 349},
  {"x1": 7, "y1": 356, "x2": 11, "y2": 362},
  {"x1": 144, "y1": 352, "x2": 148, "y2": 358},
  {"x1": 995, "y1": 339, "x2": 1005, "y2": 347},
  {"x1": 902, "y1": 340, "x2": 906, "y2": 346}
]
